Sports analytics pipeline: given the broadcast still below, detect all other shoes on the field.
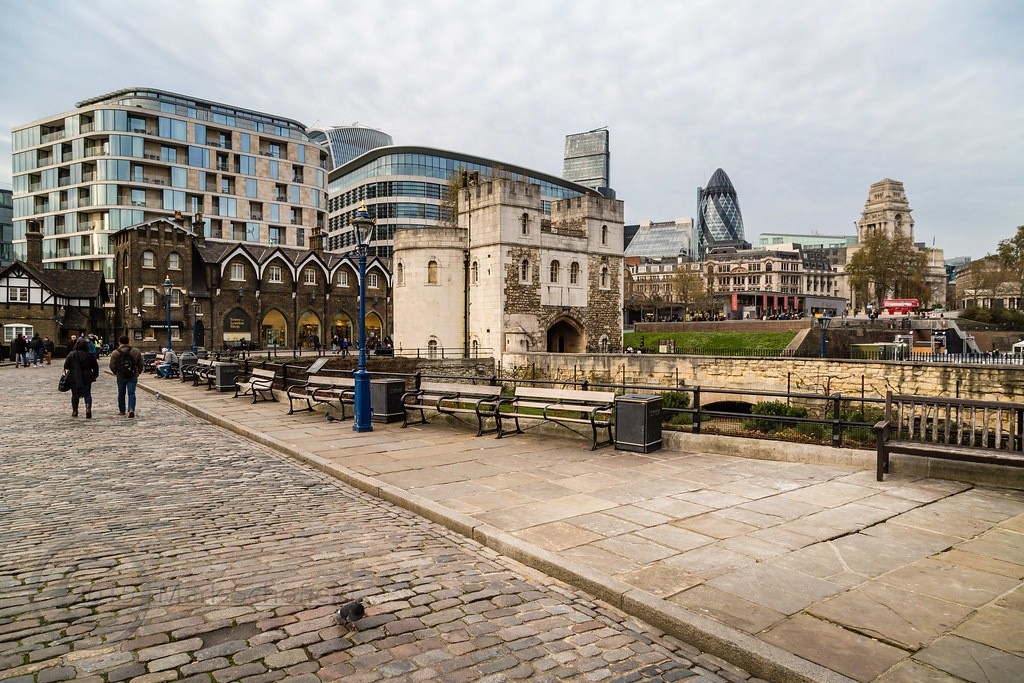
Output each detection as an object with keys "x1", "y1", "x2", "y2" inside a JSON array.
[
  {"x1": 128, "y1": 410, "x2": 134, "y2": 418},
  {"x1": 118, "y1": 411, "x2": 125, "y2": 415}
]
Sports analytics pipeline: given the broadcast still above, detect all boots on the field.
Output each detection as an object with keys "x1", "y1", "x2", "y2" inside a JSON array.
[
  {"x1": 86, "y1": 403, "x2": 91, "y2": 418},
  {"x1": 72, "y1": 403, "x2": 78, "y2": 417}
]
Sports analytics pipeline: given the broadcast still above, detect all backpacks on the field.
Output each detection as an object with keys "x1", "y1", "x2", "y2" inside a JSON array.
[{"x1": 116, "y1": 348, "x2": 137, "y2": 378}]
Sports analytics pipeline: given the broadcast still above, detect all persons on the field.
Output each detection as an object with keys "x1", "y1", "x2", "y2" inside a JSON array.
[
  {"x1": 109, "y1": 336, "x2": 143, "y2": 417},
  {"x1": 647, "y1": 308, "x2": 880, "y2": 321},
  {"x1": 155, "y1": 347, "x2": 179, "y2": 379},
  {"x1": 13, "y1": 330, "x2": 392, "y2": 369},
  {"x1": 63, "y1": 339, "x2": 99, "y2": 418}
]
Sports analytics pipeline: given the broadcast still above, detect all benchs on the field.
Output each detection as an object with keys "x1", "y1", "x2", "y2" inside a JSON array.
[
  {"x1": 374, "y1": 349, "x2": 393, "y2": 356},
  {"x1": 142, "y1": 354, "x2": 219, "y2": 391},
  {"x1": 232, "y1": 368, "x2": 279, "y2": 404},
  {"x1": 873, "y1": 391, "x2": 1024, "y2": 481},
  {"x1": 287, "y1": 365, "x2": 358, "y2": 421},
  {"x1": 400, "y1": 372, "x2": 616, "y2": 451}
]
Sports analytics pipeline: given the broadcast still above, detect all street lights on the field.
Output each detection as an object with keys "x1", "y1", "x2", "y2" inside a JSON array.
[
  {"x1": 160, "y1": 275, "x2": 174, "y2": 351},
  {"x1": 59, "y1": 306, "x2": 65, "y2": 344},
  {"x1": 349, "y1": 199, "x2": 377, "y2": 434},
  {"x1": 191, "y1": 298, "x2": 199, "y2": 356},
  {"x1": 817, "y1": 309, "x2": 831, "y2": 358}
]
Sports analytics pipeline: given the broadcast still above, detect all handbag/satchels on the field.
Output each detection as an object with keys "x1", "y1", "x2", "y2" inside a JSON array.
[{"x1": 58, "y1": 368, "x2": 75, "y2": 392}]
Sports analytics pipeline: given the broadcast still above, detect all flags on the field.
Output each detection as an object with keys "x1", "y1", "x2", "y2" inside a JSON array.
[
  {"x1": 774, "y1": 294, "x2": 778, "y2": 310},
  {"x1": 763, "y1": 293, "x2": 767, "y2": 310},
  {"x1": 793, "y1": 296, "x2": 798, "y2": 309},
  {"x1": 784, "y1": 295, "x2": 788, "y2": 310},
  {"x1": 732, "y1": 293, "x2": 737, "y2": 310}
]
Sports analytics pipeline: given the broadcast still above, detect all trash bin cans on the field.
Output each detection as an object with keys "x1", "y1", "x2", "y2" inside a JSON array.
[
  {"x1": 370, "y1": 378, "x2": 406, "y2": 424},
  {"x1": 179, "y1": 352, "x2": 198, "y2": 380},
  {"x1": 216, "y1": 363, "x2": 239, "y2": 392},
  {"x1": 615, "y1": 394, "x2": 663, "y2": 453}
]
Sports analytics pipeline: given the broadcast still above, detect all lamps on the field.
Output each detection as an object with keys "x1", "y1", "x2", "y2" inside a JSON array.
[
  {"x1": 55, "y1": 305, "x2": 65, "y2": 325},
  {"x1": 235, "y1": 287, "x2": 243, "y2": 301},
  {"x1": 371, "y1": 293, "x2": 378, "y2": 306},
  {"x1": 309, "y1": 291, "x2": 316, "y2": 303}
]
